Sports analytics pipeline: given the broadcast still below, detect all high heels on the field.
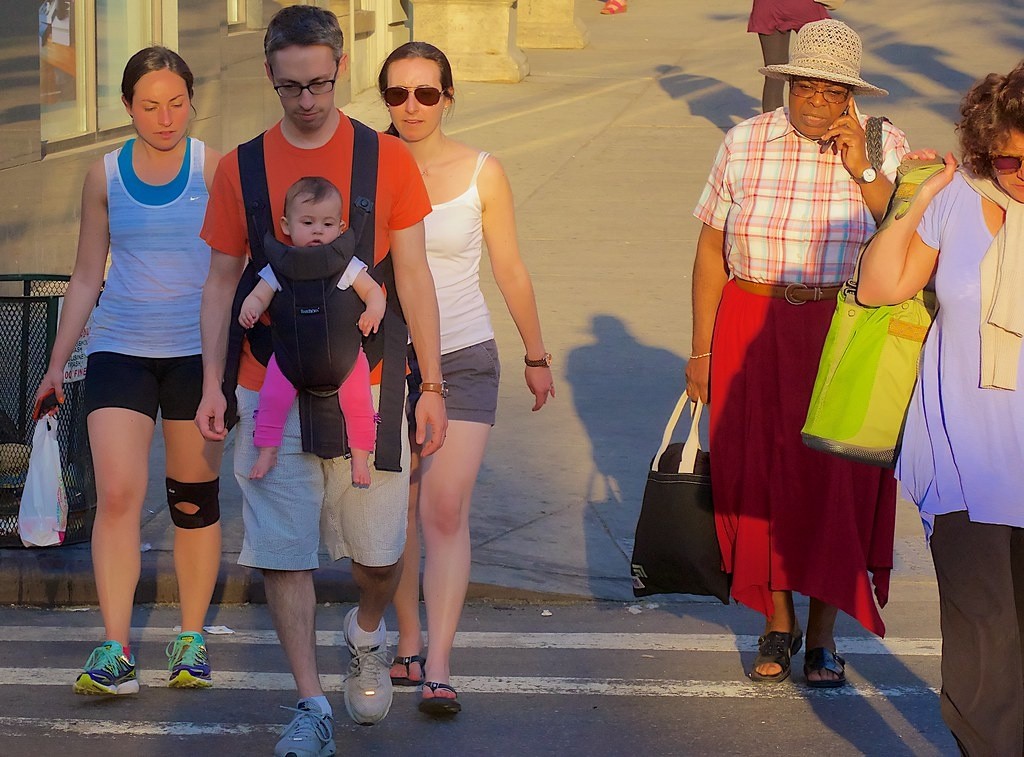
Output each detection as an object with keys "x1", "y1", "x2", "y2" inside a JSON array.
[{"x1": 600, "y1": 1, "x2": 628, "y2": 14}]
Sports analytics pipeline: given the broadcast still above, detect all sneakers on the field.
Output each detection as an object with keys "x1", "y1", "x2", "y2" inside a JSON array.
[
  {"x1": 72, "y1": 640, "x2": 139, "y2": 695},
  {"x1": 343, "y1": 605, "x2": 394, "y2": 726},
  {"x1": 274, "y1": 697, "x2": 337, "y2": 757},
  {"x1": 166, "y1": 630, "x2": 212, "y2": 688}
]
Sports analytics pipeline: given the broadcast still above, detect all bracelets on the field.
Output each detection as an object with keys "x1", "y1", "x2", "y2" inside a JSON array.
[{"x1": 689, "y1": 353, "x2": 712, "y2": 360}]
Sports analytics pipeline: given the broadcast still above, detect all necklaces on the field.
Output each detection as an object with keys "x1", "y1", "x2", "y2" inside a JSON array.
[{"x1": 417, "y1": 137, "x2": 446, "y2": 176}]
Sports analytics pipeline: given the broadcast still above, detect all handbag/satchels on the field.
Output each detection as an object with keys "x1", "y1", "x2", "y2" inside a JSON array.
[
  {"x1": 800, "y1": 163, "x2": 946, "y2": 467},
  {"x1": 18, "y1": 414, "x2": 68, "y2": 548},
  {"x1": 813, "y1": 1, "x2": 846, "y2": 11},
  {"x1": 630, "y1": 389, "x2": 732, "y2": 606}
]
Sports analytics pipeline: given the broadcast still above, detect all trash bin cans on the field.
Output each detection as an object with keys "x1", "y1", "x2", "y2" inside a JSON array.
[{"x1": 0, "y1": 273, "x2": 105, "y2": 547}]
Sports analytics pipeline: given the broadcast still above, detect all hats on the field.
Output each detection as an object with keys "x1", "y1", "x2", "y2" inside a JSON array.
[{"x1": 758, "y1": 19, "x2": 889, "y2": 97}]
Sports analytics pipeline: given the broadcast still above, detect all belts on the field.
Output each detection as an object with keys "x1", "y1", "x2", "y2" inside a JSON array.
[{"x1": 735, "y1": 275, "x2": 839, "y2": 305}]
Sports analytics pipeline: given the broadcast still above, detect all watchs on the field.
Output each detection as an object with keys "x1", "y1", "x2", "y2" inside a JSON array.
[
  {"x1": 852, "y1": 165, "x2": 878, "y2": 185},
  {"x1": 524, "y1": 353, "x2": 553, "y2": 367},
  {"x1": 419, "y1": 380, "x2": 449, "y2": 398}
]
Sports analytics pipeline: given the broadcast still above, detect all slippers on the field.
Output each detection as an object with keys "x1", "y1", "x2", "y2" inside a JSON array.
[
  {"x1": 418, "y1": 679, "x2": 462, "y2": 713},
  {"x1": 389, "y1": 655, "x2": 426, "y2": 685},
  {"x1": 751, "y1": 630, "x2": 803, "y2": 682},
  {"x1": 803, "y1": 647, "x2": 846, "y2": 687}
]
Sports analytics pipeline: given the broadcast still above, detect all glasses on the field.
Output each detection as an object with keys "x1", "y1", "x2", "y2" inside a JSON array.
[
  {"x1": 985, "y1": 151, "x2": 1024, "y2": 173},
  {"x1": 381, "y1": 87, "x2": 446, "y2": 106},
  {"x1": 790, "y1": 75, "x2": 850, "y2": 104},
  {"x1": 268, "y1": 62, "x2": 338, "y2": 99}
]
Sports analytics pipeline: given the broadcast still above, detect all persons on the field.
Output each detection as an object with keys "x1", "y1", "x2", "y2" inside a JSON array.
[
  {"x1": 686, "y1": 19, "x2": 912, "y2": 688},
  {"x1": 856, "y1": 61, "x2": 1024, "y2": 757},
  {"x1": 600, "y1": 0, "x2": 627, "y2": 15},
  {"x1": 747, "y1": 0, "x2": 833, "y2": 113},
  {"x1": 238, "y1": 176, "x2": 386, "y2": 485},
  {"x1": 378, "y1": 42, "x2": 555, "y2": 712},
  {"x1": 194, "y1": 4, "x2": 448, "y2": 757},
  {"x1": 34, "y1": 46, "x2": 250, "y2": 695}
]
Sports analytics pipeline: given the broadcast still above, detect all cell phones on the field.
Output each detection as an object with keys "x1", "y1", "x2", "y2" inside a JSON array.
[
  {"x1": 820, "y1": 105, "x2": 850, "y2": 153},
  {"x1": 33, "y1": 388, "x2": 58, "y2": 421}
]
